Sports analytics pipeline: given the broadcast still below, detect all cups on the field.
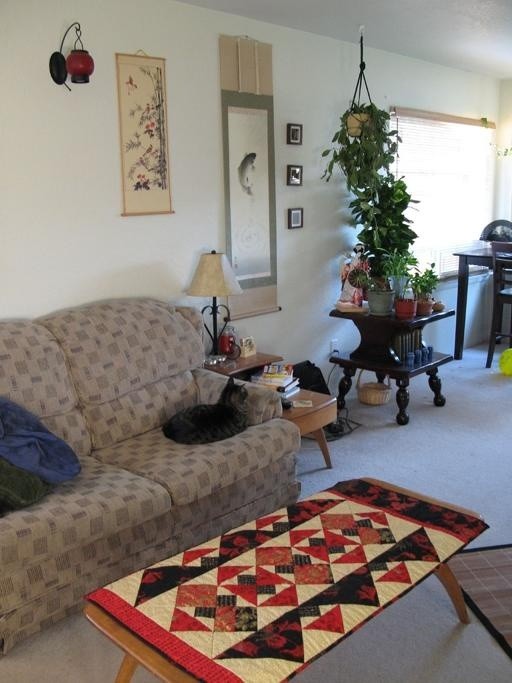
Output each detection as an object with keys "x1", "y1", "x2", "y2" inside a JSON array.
[
  {"x1": 415, "y1": 345, "x2": 432, "y2": 363},
  {"x1": 406, "y1": 351, "x2": 414, "y2": 365}
]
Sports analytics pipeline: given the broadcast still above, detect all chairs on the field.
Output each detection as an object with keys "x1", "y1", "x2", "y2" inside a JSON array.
[
  {"x1": 479, "y1": 219, "x2": 512, "y2": 245},
  {"x1": 485, "y1": 240, "x2": 512, "y2": 369}
]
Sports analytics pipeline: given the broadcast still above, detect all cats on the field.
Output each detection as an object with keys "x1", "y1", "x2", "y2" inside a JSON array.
[{"x1": 162, "y1": 376, "x2": 251, "y2": 444}]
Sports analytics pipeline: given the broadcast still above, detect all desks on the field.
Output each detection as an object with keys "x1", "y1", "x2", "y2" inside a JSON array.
[
  {"x1": 327, "y1": 307, "x2": 457, "y2": 425},
  {"x1": 203, "y1": 349, "x2": 285, "y2": 377},
  {"x1": 282, "y1": 390, "x2": 340, "y2": 470},
  {"x1": 452, "y1": 247, "x2": 512, "y2": 360}
]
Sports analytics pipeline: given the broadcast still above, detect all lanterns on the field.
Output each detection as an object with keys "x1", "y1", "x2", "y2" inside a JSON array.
[{"x1": 65, "y1": 49, "x2": 95, "y2": 83}]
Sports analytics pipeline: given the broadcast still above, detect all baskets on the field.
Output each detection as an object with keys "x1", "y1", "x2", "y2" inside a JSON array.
[{"x1": 356, "y1": 368, "x2": 392, "y2": 406}]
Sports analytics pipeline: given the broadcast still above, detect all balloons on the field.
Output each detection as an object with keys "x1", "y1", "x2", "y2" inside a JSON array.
[{"x1": 499, "y1": 347, "x2": 512, "y2": 375}]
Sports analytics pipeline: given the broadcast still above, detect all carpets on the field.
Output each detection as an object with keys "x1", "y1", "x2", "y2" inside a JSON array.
[{"x1": 446, "y1": 543, "x2": 511, "y2": 662}]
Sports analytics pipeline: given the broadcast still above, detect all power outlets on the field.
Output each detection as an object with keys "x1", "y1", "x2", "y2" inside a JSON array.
[{"x1": 329, "y1": 337, "x2": 342, "y2": 356}]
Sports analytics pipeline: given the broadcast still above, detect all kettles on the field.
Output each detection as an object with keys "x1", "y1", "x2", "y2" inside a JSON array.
[{"x1": 219, "y1": 325, "x2": 236, "y2": 353}]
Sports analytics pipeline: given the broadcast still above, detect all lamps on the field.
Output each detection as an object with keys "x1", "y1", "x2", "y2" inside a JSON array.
[
  {"x1": 185, "y1": 248, "x2": 243, "y2": 364},
  {"x1": 49, "y1": 17, "x2": 98, "y2": 93}
]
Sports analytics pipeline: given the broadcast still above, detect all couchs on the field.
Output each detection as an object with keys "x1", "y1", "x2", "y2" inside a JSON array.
[{"x1": 0, "y1": 295, "x2": 305, "y2": 660}]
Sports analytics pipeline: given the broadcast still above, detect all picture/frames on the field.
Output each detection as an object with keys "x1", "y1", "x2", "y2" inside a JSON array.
[
  {"x1": 285, "y1": 122, "x2": 304, "y2": 146},
  {"x1": 287, "y1": 207, "x2": 304, "y2": 229},
  {"x1": 285, "y1": 163, "x2": 303, "y2": 187}
]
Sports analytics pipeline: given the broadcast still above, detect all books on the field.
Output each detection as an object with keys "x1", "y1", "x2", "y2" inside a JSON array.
[
  {"x1": 249, "y1": 367, "x2": 301, "y2": 398},
  {"x1": 335, "y1": 300, "x2": 365, "y2": 313}
]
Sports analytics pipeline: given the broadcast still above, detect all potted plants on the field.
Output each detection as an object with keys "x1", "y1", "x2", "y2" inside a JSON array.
[{"x1": 319, "y1": 20, "x2": 447, "y2": 320}]
